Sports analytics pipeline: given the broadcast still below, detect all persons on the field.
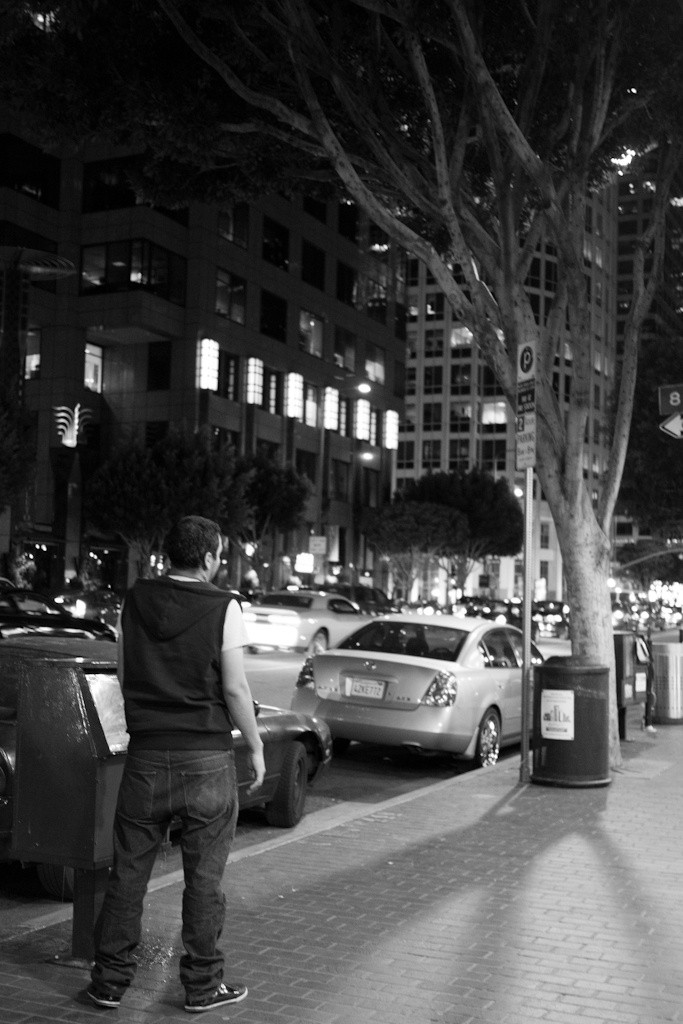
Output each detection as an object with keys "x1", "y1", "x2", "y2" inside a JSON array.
[
  {"x1": 70, "y1": 563, "x2": 96, "y2": 595},
  {"x1": 0, "y1": 553, "x2": 28, "y2": 590},
  {"x1": 87, "y1": 517, "x2": 266, "y2": 1010}
]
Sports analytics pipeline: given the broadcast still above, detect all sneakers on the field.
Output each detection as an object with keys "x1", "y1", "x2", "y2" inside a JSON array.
[
  {"x1": 87, "y1": 983, "x2": 121, "y2": 1008},
  {"x1": 184, "y1": 984, "x2": 248, "y2": 1012}
]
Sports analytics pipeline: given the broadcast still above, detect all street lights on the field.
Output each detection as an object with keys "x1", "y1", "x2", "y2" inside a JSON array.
[{"x1": 313, "y1": 381, "x2": 374, "y2": 533}]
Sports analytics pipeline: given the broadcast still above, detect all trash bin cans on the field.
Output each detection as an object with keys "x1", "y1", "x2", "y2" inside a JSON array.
[
  {"x1": 650, "y1": 642, "x2": 683, "y2": 725},
  {"x1": 613, "y1": 632, "x2": 636, "y2": 740},
  {"x1": 8, "y1": 656, "x2": 129, "y2": 960},
  {"x1": 533, "y1": 654, "x2": 612, "y2": 788}
]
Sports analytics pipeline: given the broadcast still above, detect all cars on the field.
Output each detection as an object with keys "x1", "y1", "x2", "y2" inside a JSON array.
[
  {"x1": 0, "y1": 575, "x2": 659, "y2": 902},
  {"x1": 313, "y1": 583, "x2": 402, "y2": 617},
  {"x1": 246, "y1": 589, "x2": 374, "y2": 658},
  {"x1": 289, "y1": 614, "x2": 545, "y2": 770}
]
